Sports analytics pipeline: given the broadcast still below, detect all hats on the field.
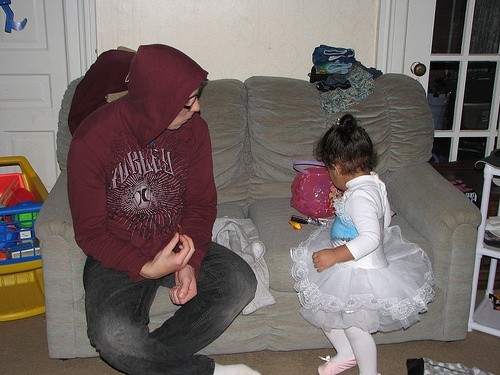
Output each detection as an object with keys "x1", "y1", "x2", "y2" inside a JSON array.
[{"x1": 475, "y1": 148, "x2": 500, "y2": 187}]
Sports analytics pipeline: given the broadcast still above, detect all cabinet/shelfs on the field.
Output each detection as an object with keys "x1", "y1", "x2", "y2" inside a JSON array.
[{"x1": 468, "y1": 152, "x2": 500, "y2": 338}]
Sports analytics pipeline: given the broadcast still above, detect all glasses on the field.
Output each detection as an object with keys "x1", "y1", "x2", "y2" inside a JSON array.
[{"x1": 183, "y1": 93, "x2": 200, "y2": 109}]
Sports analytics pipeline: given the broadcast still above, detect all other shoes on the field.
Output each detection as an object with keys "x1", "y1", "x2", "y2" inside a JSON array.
[{"x1": 318, "y1": 355, "x2": 358, "y2": 375}]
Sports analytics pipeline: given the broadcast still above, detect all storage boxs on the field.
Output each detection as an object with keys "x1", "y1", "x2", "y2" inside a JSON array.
[{"x1": 0, "y1": 156, "x2": 49, "y2": 321}]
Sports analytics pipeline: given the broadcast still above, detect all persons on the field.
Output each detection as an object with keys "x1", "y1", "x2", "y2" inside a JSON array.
[
  {"x1": 288, "y1": 113, "x2": 435, "y2": 375},
  {"x1": 67, "y1": 44, "x2": 264, "y2": 375}
]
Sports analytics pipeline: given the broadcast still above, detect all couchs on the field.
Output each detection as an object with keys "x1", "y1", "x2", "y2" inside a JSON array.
[{"x1": 34, "y1": 73, "x2": 482, "y2": 361}]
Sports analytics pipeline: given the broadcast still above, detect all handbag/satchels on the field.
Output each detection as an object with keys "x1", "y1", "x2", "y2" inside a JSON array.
[{"x1": 290, "y1": 160, "x2": 343, "y2": 218}]
